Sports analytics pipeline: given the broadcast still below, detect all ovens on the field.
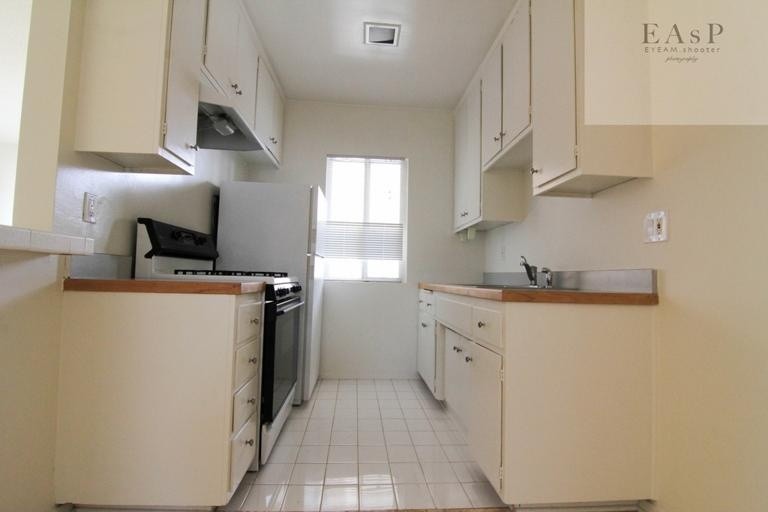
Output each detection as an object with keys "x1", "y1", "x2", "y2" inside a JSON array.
[{"x1": 258, "y1": 296, "x2": 306, "y2": 467}]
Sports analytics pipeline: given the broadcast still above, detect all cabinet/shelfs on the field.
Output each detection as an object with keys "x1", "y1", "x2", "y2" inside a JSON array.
[
  {"x1": 482, "y1": 14, "x2": 532, "y2": 173},
  {"x1": 531, "y1": 1, "x2": 654, "y2": 196},
  {"x1": 258, "y1": 57, "x2": 286, "y2": 169},
  {"x1": 73, "y1": 0, "x2": 207, "y2": 174},
  {"x1": 206, "y1": 0, "x2": 257, "y2": 132},
  {"x1": 436, "y1": 292, "x2": 655, "y2": 504},
  {"x1": 60, "y1": 281, "x2": 262, "y2": 504},
  {"x1": 453, "y1": 82, "x2": 521, "y2": 233},
  {"x1": 417, "y1": 286, "x2": 436, "y2": 400}
]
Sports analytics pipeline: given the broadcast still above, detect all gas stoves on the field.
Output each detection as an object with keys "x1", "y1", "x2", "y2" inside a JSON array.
[{"x1": 153, "y1": 267, "x2": 288, "y2": 279}]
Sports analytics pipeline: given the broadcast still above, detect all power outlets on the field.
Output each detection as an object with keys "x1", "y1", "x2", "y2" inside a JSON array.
[
  {"x1": 644, "y1": 211, "x2": 667, "y2": 242},
  {"x1": 84, "y1": 192, "x2": 97, "y2": 224}
]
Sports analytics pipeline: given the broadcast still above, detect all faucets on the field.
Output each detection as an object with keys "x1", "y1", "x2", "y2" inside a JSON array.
[
  {"x1": 540, "y1": 266, "x2": 554, "y2": 289},
  {"x1": 520, "y1": 254, "x2": 538, "y2": 289}
]
